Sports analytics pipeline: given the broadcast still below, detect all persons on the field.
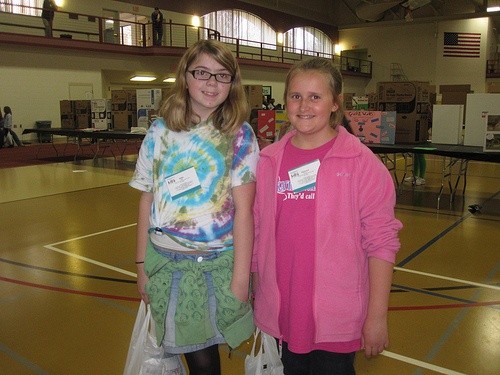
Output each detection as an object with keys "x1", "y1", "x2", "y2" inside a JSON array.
[
  {"x1": 0, "y1": 106, "x2": 14, "y2": 148},
  {"x1": 151, "y1": 7, "x2": 163, "y2": 47},
  {"x1": 128, "y1": 39, "x2": 261, "y2": 375},
  {"x1": 250, "y1": 57, "x2": 402, "y2": 375},
  {"x1": 42, "y1": 0, "x2": 58, "y2": 38}
]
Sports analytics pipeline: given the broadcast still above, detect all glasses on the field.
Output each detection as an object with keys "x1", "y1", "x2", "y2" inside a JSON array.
[{"x1": 187, "y1": 70, "x2": 234, "y2": 84}]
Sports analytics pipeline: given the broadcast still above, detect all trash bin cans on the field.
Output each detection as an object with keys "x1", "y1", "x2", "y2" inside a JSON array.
[{"x1": 36, "y1": 120, "x2": 51, "y2": 142}]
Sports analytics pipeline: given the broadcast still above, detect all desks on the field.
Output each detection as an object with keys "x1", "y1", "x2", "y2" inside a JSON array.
[
  {"x1": 364, "y1": 142, "x2": 500, "y2": 221},
  {"x1": 24, "y1": 126, "x2": 144, "y2": 166}
]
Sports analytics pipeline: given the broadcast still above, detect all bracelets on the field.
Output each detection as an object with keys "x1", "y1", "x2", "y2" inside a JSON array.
[{"x1": 135, "y1": 261, "x2": 144, "y2": 264}]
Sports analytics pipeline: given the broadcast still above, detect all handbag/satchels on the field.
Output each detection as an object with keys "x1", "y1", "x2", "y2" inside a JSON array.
[
  {"x1": 245, "y1": 328, "x2": 284, "y2": 375},
  {"x1": 122, "y1": 296, "x2": 186, "y2": 375}
]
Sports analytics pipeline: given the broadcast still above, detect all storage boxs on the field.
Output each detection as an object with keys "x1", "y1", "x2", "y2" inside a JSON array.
[
  {"x1": 59, "y1": 85, "x2": 174, "y2": 130},
  {"x1": 242, "y1": 57, "x2": 500, "y2": 141}
]
